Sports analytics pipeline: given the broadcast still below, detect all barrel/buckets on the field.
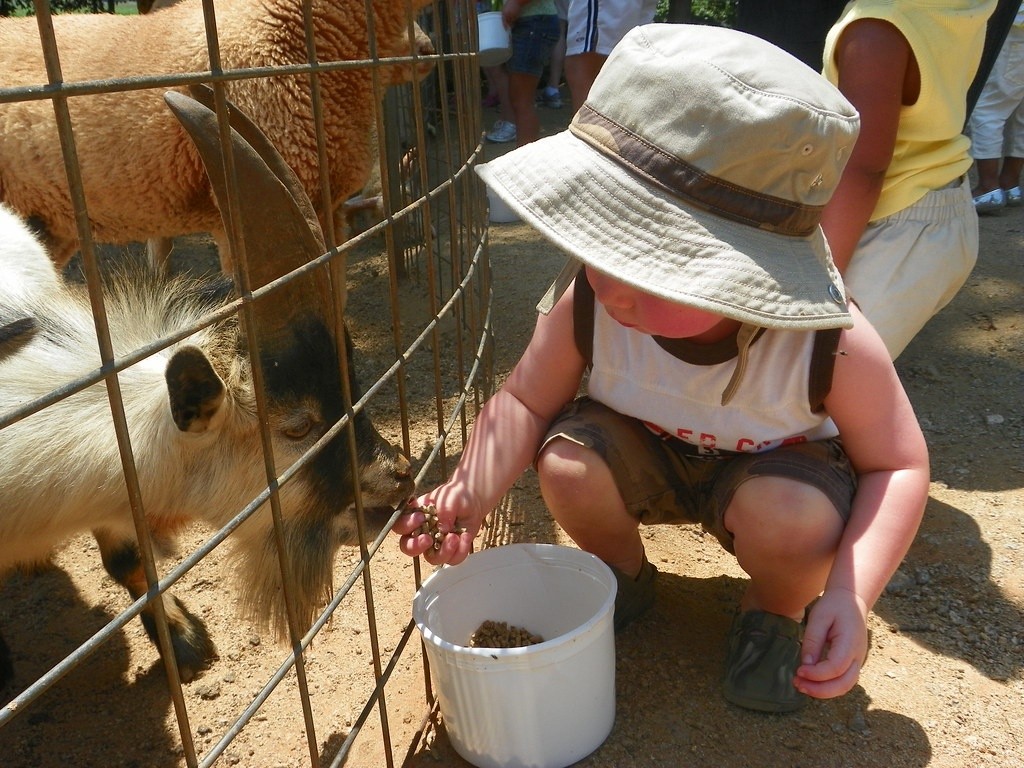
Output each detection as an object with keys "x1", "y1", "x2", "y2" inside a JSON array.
[
  {"x1": 468, "y1": 11, "x2": 513, "y2": 67},
  {"x1": 412, "y1": 544, "x2": 618, "y2": 768}
]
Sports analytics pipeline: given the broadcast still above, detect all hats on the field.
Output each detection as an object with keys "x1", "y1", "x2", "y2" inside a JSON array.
[{"x1": 474, "y1": 23, "x2": 854, "y2": 406}]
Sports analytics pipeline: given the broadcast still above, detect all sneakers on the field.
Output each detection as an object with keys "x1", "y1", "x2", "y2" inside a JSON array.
[
  {"x1": 485, "y1": 118, "x2": 518, "y2": 142},
  {"x1": 496, "y1": 102, "x2": 505, "y2": 112},
  {"x1": 543, "y1": 86, "x2": 563, "y2": 109}
]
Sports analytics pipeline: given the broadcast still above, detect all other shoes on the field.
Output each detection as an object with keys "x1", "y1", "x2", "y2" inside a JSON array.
[
  {"x1": 973, "y1": 188, "x2": 1005, "y2": 213},
  {"x1": 482, "y1": 93, "x2": 499, "y2": 107},
  {"x1": 1003, "y1": 186, "x2": 1022, "y2": 206}
]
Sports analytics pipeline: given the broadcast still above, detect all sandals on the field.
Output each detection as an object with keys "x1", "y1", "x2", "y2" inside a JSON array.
[
  {"x1": 605, "y1": 548, "x2": 658, "y2": 634},
  {"x1": 721, "y1": 597, "x2": 814, "y2": 714}
]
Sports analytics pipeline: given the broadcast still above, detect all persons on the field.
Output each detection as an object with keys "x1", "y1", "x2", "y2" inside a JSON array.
[
  {"x1": 812, "y1": 1, "x2": 996, "y2": 374},
  {"x1": 392, "y1": 19, "x2": 932, "y2": 720},
  {"x1": 466, "y1": 0, "x2": 659, "y2": 228},
  {"x1": 964, "y1": 0, "x2": 1024, "y2": 214}
]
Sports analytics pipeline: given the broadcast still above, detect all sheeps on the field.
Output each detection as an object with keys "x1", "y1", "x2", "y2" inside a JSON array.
[
  {"x1": 0, "y1": 0, "x2": 434, "y2": 319},
  {"x1": 0, "y1": 83, "x2": 415, "y2": 684}
]
